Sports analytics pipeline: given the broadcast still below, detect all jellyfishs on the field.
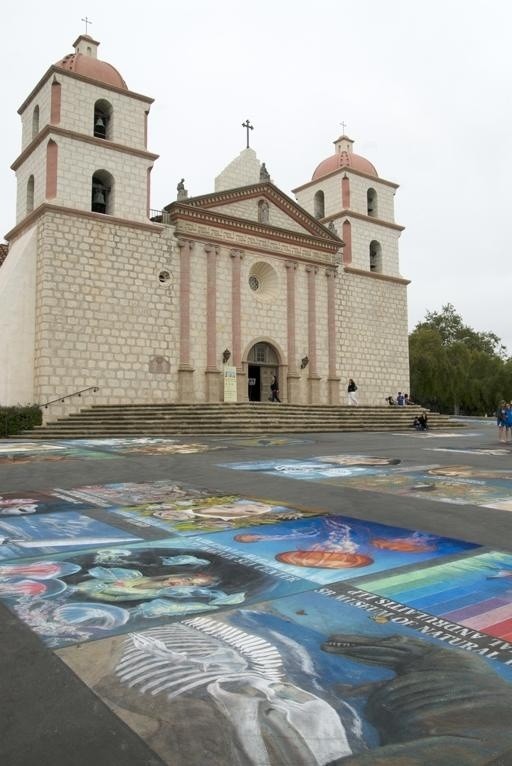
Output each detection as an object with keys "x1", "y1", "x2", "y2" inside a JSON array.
[
  {"x1": 0, "y1": 548, "x2": 246, "y2": 649},
  {"x1": 233, "y1": 517, "x2": 440, "y2": 571}
]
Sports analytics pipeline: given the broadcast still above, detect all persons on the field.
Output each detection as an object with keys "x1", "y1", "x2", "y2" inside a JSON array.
[
  {"x1": 413, "y1": 416, "x2": 422, "y2": 431},
  {"x1": 269, "y1": 375, "x2": 281, "y2": 402},
  {"x1": 347, "y1": 378, "x2": 358, "y2": 406},
  {"x1": 176, "y1": 177, "x2": 185, "y2": 190},
  {"x1": 419, "y1": 410, "x2": 430, "y2": 431},
  {"x1": 259, "y1": 161, "x2": 269, "y2": 178},
  {"x1": 495, "y1": 398, "x2": 512, "y2": 443},
  {"x1": 260, "y1": 199, "x2": 268, "y2": 222},
  {"x1": 404, "y1": 393, "x2": 415, "y2": 404},
  {"x1": 396, "y1": 391, "x2": 405, "y2": 405},
  {"x1": 388, "y1": 395, "x2": 396, "y2": 405},
  {"x1": 328, "y1": 218, "x2": 335, "y2": 230}
]
